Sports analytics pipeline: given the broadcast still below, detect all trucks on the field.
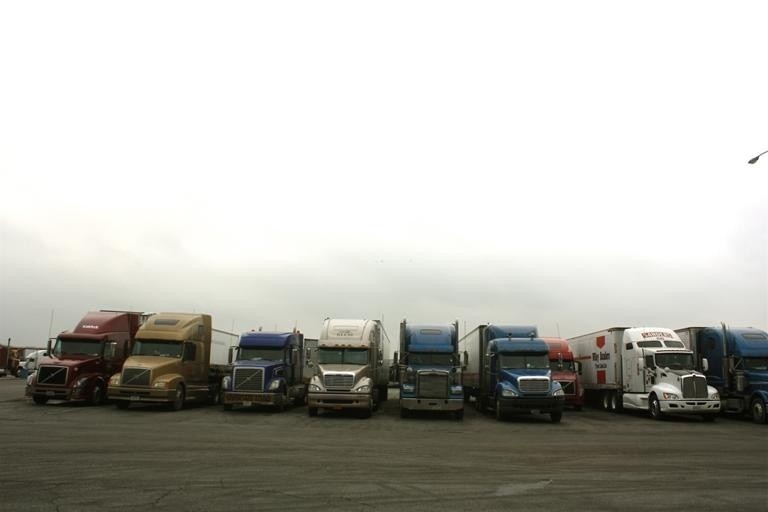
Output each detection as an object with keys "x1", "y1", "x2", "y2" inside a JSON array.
[
  {"x1": 0, "y1": 345, "x2": 48, "y2": 377},
  {"x1": 223, "y1": 330, "x2": 323, "y2": 412},
  {"x1": 26, "y1": 311, "x2": 152, "y2": 406},
  {"x1": 395, "y1": 320, "x2": 467, "y2": 418},
  {"x1": 672, "y1": 326, "x2": 768, "y2": 425},
  {"x1": 310, "y1": 319, "x2": 392, "y2": 415},
  {"x1": 527, "y1": 337, "x2": 584, "y2": 414},
  {"x1": 110, "y1": 312, "x2": 240, "y2": 410},
  {"x1": 575, "y1": 327, "x2": 723, "y2": 418},
  {"x1": 450, "y1": 324, "x2": 566, "y2": 423}
]
